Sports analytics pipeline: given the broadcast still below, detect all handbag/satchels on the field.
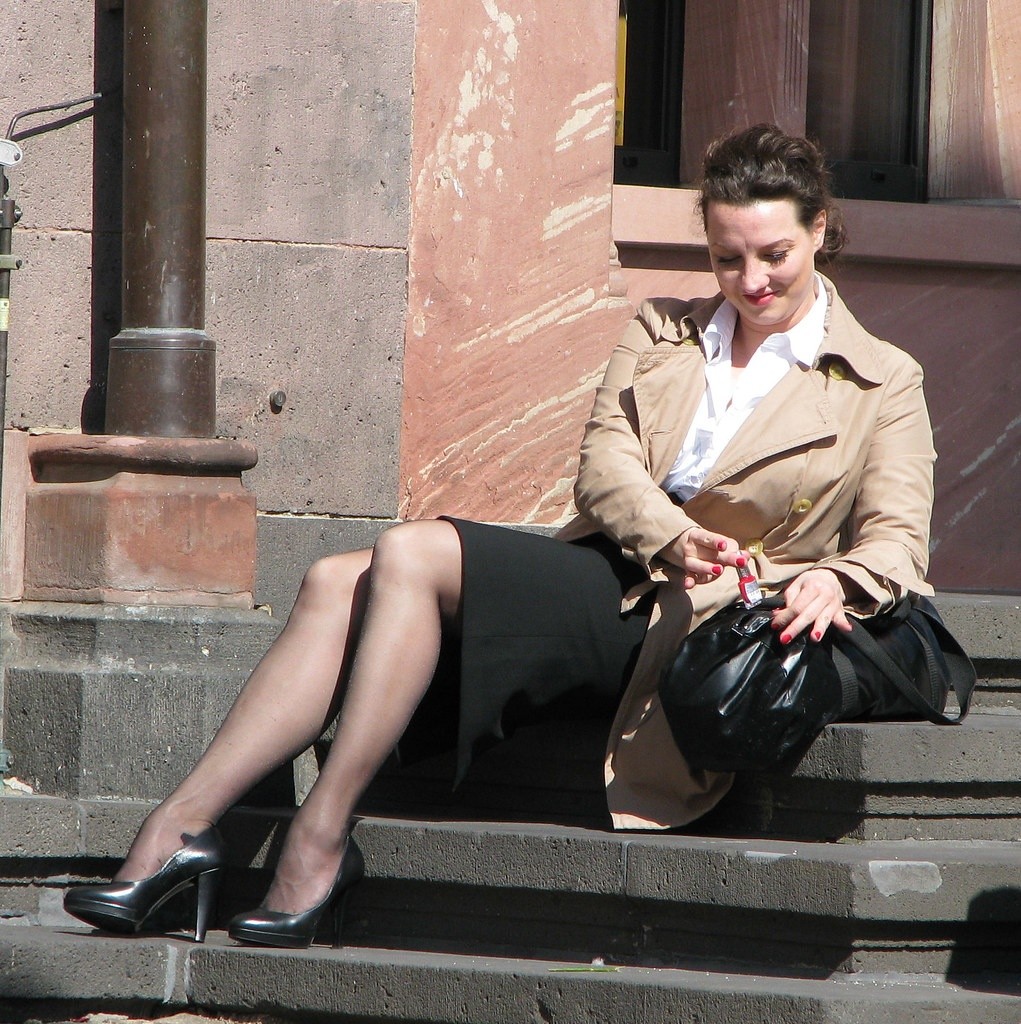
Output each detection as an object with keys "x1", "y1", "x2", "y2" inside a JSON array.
[{"x1": 657, "y1": 594, "x2": 977, "y2": 775}]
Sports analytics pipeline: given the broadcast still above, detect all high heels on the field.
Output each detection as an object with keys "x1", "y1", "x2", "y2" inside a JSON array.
[
  {"x1": 63, "y1": 826, "x2": 226, "y2": 943},
  {"x1": 229, "y1": 833, "x2": 364, "y2": 948}
]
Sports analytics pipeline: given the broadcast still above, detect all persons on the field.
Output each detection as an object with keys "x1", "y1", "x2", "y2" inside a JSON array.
[{"x1": 64, "y1": 124, "x2": 938, "y2": 946}]
